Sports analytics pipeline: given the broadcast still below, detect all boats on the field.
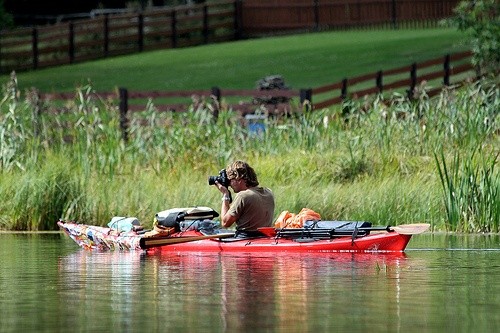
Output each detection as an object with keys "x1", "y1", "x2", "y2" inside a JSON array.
[{"x1": 58, "y1": 206, "x2": 413, "y2": 256}]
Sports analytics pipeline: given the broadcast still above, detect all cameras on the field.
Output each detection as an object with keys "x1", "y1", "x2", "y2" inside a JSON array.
[{"x1": 209, "y1": 169, "x2": 228, "y2": 191}]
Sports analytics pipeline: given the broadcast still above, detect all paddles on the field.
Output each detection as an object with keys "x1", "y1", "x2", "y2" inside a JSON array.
[
  {"x1": 334, "y1": 222, "x2": 431, "y2": 235},
  {"x1": 139, "y1": 232, "x2": 247, "y2": 250}
]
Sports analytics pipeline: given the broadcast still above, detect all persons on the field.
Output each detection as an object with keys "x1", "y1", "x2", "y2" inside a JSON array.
[{"x1": 214, "y1": 161, "x2": 275, "y2": 230}]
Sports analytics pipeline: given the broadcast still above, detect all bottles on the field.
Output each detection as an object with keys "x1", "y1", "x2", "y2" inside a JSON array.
[{"x1": 179, "y1": 219, "x2": 221, "y2": 232}]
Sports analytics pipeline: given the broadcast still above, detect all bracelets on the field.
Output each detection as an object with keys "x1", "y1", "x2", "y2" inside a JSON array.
[{"x1": 221, "y1": 196, "x2": 230, "y2": 201}]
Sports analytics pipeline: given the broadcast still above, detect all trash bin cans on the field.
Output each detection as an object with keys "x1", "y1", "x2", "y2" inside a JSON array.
[{"x1": 246, "y1": 114, "x2": 266, "y2": 141}]
